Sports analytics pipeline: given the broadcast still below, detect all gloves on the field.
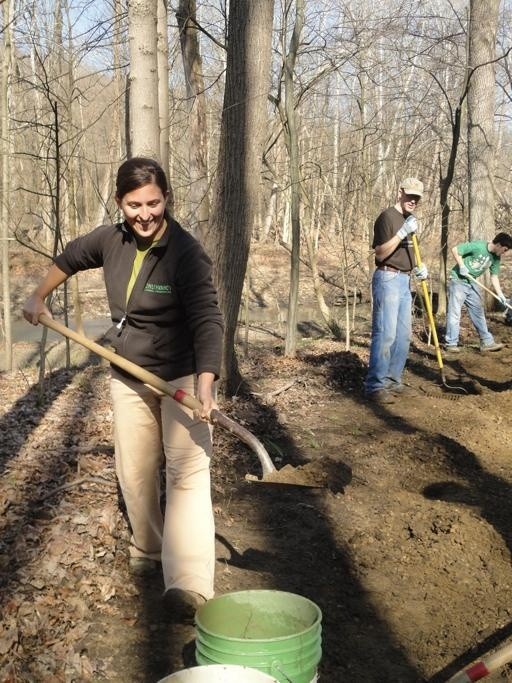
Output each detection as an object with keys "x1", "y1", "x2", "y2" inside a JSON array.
[
  {"x1": 396, "y1": 216, "x2": 418, "y2": 240},
  {"x1": 458, "y1": 265, "x2": 468, "y2": 276},
  {"x1": 498, "y1": 293, "x2": 506, "y2": 305},
  {"x1": 414, "y1": 264, "x2": 428, "y2": 280}
]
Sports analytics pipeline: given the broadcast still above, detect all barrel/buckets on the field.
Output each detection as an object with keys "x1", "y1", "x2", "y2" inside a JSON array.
[
  {"x1": 195, "y1": 589, "x2": 323, "y2": 683},
  {"x1": 157, "y1": 664, "x2": 280, "y2": 683}
]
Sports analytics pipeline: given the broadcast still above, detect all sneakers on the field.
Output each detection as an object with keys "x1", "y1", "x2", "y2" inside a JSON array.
[
  {"x1": 164, "y1": 589, "x2": 207, "y2": 624},
  {"x1": 362, "y1": 386, "x2": 416, "y2": 404},
  {"x1": 479, "y1": 343, "x2": 502, "y2": 352},
  {"x1": 446, "y1": 346, "x2": 459, "y2": 353},
  {"x1": 129, "y1": 557, "x2": 155, "y2": 574}
]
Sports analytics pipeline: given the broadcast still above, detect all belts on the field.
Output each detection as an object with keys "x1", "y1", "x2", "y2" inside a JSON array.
[{"x1": 379, "y1": 266, "x2": 410, "y2": 275}]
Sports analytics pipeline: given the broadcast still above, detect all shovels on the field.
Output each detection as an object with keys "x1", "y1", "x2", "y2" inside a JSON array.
[{"x1": 36, "y1": 312, "x2": 336, "y2": 489}]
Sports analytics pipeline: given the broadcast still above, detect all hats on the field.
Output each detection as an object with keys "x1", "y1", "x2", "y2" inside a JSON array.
[{"x1": 401, "y1": 178, "x2": 423, "y2": 198}]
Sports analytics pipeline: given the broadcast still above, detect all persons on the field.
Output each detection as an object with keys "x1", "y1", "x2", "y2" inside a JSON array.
[
  {"x1": 363, "y1": 177, "x2": 428, "y2": 404},
  {"x1": 23, "y1": 158, "x2": 225, "y2": 627},
  {"x1": 444, "y1": 232, "x2": 512, "y2": 352}
]
